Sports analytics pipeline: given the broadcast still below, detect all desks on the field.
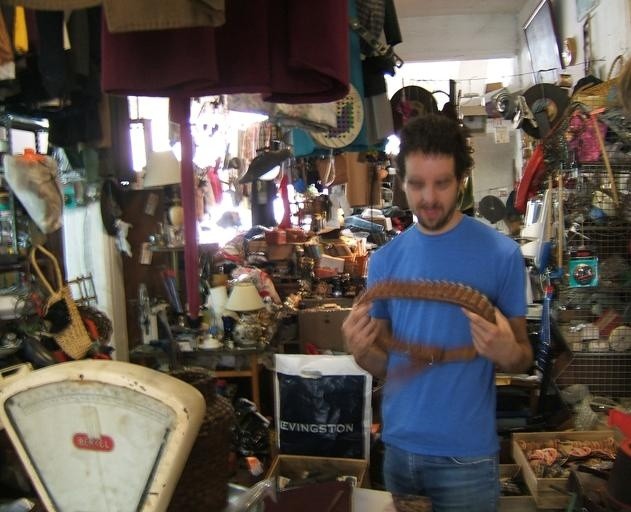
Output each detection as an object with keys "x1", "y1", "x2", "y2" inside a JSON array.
[{"x1": 136, "y1": 347, "x2": 261, "y2": 413}]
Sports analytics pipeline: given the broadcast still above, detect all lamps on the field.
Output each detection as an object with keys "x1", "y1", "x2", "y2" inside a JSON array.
[
  {"x1": 143, "y1": 151, "x2": 185, "y2": 225},
  {"x1": 238, "y1": 148, "x2": 290, "y2": 181},
  {"x1": 225, "y1": 282, "x2": 267, "y2": 348}
]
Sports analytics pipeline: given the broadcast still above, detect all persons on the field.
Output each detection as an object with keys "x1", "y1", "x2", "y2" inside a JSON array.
[{"x1": 341, "y1": 111, "x2": 533, "y2": 512}]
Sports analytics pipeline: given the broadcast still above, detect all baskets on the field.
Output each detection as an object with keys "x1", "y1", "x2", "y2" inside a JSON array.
[{"x1": 46, "y1": 287, "x2": 91, "y2": 360}]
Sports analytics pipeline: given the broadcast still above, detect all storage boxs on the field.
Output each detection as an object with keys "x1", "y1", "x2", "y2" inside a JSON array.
[
  {"x1": 298, "y1": 297, "x2": 358, "y2": 352},
  {"x1": 265, "y1": 454, "x2": 370, "y2": 488},
  {"x1": 510, "y1": 430, "x2": 626, "y2": 509}
]
